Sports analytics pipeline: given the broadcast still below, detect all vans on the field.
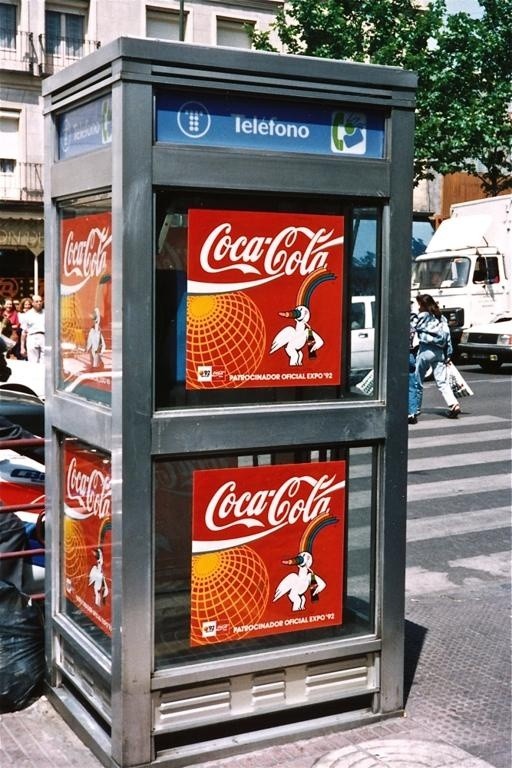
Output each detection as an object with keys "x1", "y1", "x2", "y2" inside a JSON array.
[{"x1": 349, "y1": 296, "x2": 375, "y2": 371}]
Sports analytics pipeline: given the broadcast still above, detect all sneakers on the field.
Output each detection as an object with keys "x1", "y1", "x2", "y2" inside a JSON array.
[{"x1": 408, "y1": 411, "x2": 421, "y2": 424}]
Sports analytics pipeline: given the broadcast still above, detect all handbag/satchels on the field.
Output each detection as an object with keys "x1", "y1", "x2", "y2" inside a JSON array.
[{"x1": 446, "y1": 361, "x2": 474, "y2": 398}]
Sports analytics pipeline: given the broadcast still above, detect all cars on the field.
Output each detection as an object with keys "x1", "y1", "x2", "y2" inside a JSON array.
[
  {"x1": 456, "y1": 321, "x2": 512, "y2": 370},
  {"x1": 0, "y1": 383, "x2": 44, "y2": 464}
]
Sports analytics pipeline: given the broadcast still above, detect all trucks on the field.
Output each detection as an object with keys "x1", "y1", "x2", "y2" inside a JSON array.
[{"x1": 410, "y1": 194, "x2": 512, "y2": 340}]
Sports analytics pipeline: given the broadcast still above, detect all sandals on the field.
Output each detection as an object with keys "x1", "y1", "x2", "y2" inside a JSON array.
[{"x1": 449, "y1": 405, "x2": 461, "y2": 418}]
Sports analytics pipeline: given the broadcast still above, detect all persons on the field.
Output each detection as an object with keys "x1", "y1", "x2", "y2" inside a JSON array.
[
  {"x1": 408, "y1": 294, "x2": 460, "y2": 424},
  {"x1": 0, "y1": 295, "x2": 45, "y2": 381}
]
